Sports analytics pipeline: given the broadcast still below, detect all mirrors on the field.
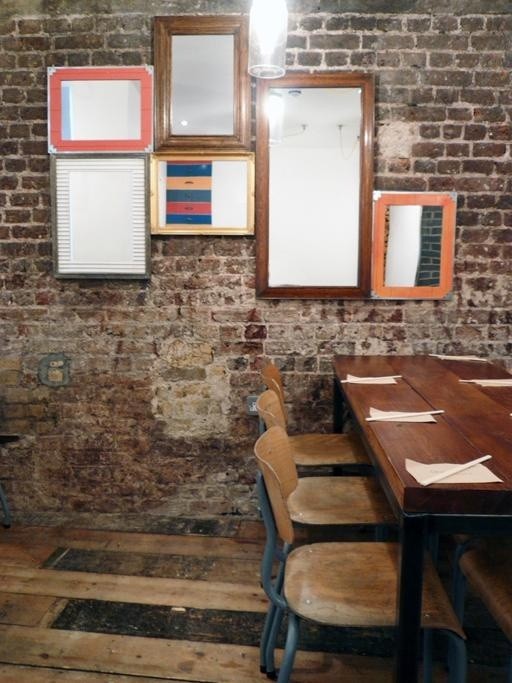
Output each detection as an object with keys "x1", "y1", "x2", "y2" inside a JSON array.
[
  {"x1": 153, "y1": 9, "x2": 252, "y2": 155},
  {"x1": 253, "y1": 66, "x2": 376, "y2": 303},
  {"x1": 371, "y1": 187, "x2": 460, "y2": 299}
]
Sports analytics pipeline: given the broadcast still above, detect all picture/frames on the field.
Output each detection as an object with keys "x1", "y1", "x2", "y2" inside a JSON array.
[
  {"x1": 146, "y1": 149, "x2": 255, "y2": 237},
  {"x1": 45, "y1": 64, "x2": 153, "y2": 282}
]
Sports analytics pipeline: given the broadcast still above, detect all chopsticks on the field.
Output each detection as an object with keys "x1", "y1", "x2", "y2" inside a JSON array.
[
  {"x1": 340, "y1": 374, "x2": 402, "y2": 383},
  {"x1": 364, "y1": 410, "x2": 445, "y2": 422},
  {"x1": 421, "y1": 454, "x2": 496, "y2": 487}
]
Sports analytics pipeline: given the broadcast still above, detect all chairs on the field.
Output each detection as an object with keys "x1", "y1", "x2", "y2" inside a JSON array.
[
  {"x1": 255, "y1": 390, "x2": 398, "y2": 680},
  {"x1": 455, "y1": 546, "x2": 511, "y2": 646},
  {"x1": 259, "y1": 361, "x2": 377, "y2": 476},
  {"x1": 251, "y1": 424, "x2": 472, "y2": 682}
]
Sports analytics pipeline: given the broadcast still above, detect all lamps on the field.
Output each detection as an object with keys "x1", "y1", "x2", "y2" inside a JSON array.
[{"x1": 247, "y1": 0, "x2": 290, "y2": 79}]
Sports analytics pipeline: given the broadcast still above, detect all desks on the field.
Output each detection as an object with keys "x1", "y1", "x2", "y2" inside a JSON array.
[{"x1": 330, "y1": 352, "x2": 512, "y2": 682}]
